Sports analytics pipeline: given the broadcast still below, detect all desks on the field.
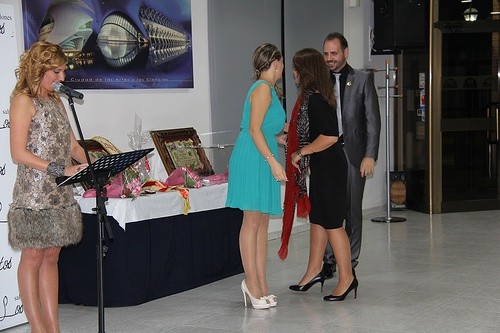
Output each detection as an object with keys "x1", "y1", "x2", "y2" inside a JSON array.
[{"x1": 57, "y1": 177, "x2": 244, "y2": 307}]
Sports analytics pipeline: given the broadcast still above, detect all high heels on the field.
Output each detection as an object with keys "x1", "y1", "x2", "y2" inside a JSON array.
[
  {"x1": 288, "y1": 270, "x2": 325, "y2": 293},
  {"x1": 323, "y1": 277, "x2": 358, "y2": 301},
  {"x1": 264, "y1": 295, "x2": 278, "y2": 307},
  {"x1": 241, "y1": 278, "x2": 271, "y2": 310}
]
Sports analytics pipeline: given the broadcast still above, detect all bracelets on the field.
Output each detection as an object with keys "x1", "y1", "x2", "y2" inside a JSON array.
[
  {"x1": 47, "y1": 162, "x2": 66, "y2": 177},
  {"x1": 266, "y1": 154, "x2": 274, "y2": 159},
  {"x1": 297, "y1": 149, "x2": 304, "y2": 158}
]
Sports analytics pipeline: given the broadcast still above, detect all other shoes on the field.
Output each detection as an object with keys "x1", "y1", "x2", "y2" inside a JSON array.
[{"x1": 320, "y1": 262, "x2": 334, "y2": 279}]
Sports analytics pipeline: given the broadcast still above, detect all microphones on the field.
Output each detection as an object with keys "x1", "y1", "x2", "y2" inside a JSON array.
[{"x1": 53, "y1": 82, "x2": 83, "y2": 99}]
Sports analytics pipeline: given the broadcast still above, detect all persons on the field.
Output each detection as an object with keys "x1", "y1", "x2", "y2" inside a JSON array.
[
  {"x1": 8, "y1": 42, "x2": 95, "y2": 333},
  {"x1": 278, "y1": 49, "x2": 358, "y2": 301},
  {"x1": 226, "y1": 43, "x2": 289, "y2": 309},
  {"x1": 322, "y1": 32, "x2": 382, "y2": 278}
]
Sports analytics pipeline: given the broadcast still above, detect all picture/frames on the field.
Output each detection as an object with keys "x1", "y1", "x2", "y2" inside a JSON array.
[{"x1": 152, "y1": 127, "x2": 215, "y2": 178}]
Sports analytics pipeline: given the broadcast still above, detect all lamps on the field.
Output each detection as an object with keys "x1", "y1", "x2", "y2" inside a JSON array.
[{"x1": 462, "y1": 1, "x2": 478, "y2": 21}]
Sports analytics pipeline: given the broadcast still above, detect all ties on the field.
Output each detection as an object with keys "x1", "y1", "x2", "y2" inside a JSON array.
[{"x1": 332, "y1": 73, "x2": 343, "y2": 137}]
